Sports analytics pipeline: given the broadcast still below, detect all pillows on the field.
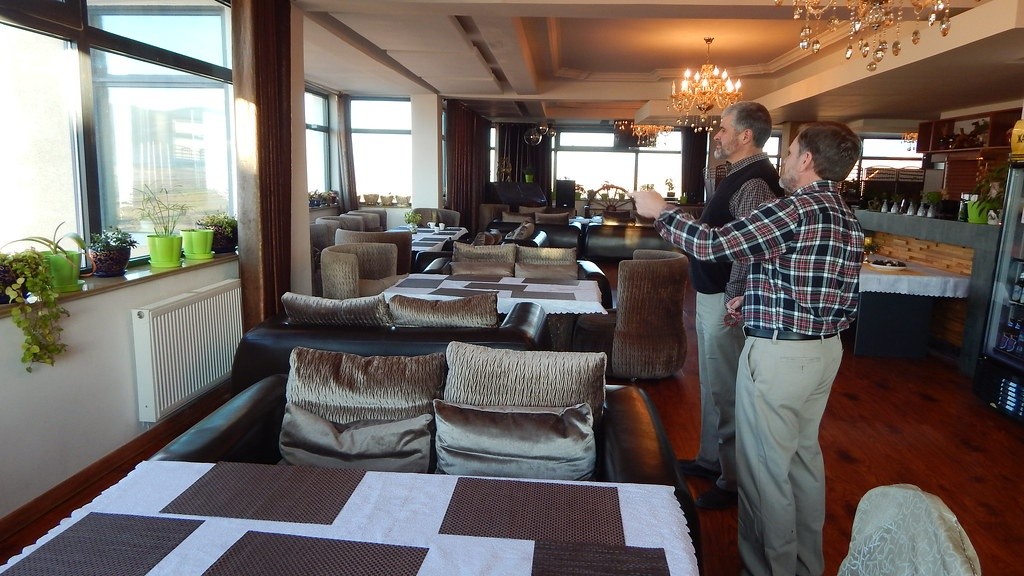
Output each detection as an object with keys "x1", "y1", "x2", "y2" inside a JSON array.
[
  {"x1": 445, "y1": 340, "x2": 607, "y2": 427},
  {"x1": 515, "y1": 244, "x2": 578, "y2": 263},
  {"x1": 502, "y1": 211, "x2": 533, "y2": 223},
  {"x1": 448, "y1": 261, "x2": 513, "y2": 277},
  {"x1": 514, "y1": 262, "x2": 579, "y2": 277},
  {"x1": 281, "y1": 403, "x2": 436, "y2": 474},
  {"x1": 451, "y1": 243, "x2": 514, "y2": 262},
  {"x1": 389, "y1": 292, "x2": 499, "y2": 327},
  {"x1": 282, "y1": 291, "x2": 397, "y2": 326},
  {"x1": 284, "y1": 347, "x2": 449, "y2": 427},
  {"x1": 519, "y1": 205, "x2": 548, "y2": 212},
  {"x1": 429, "y1": 400, "x2": 601, "y2": 480},
  {"x1": 534, "y1": 212, "x2": 569, "y2": 224}
]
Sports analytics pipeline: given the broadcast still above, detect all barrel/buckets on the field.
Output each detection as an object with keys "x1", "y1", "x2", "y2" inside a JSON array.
[{"x1": 964, "y1": 201, "x2": 996, "y2": 223}]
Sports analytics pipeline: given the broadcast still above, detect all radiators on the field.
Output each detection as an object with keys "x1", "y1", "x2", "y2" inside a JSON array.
[{"x1": 132, "y1": 278, "x2": 248, "y2": 422}]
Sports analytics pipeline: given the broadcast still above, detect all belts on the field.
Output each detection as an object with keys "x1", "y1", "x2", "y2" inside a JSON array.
[{"x1": 744, "y1": 327, "x2": 837, "y2": 340}]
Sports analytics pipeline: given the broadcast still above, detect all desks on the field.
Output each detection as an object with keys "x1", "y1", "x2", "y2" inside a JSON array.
[
  {"x1": 0, "y1": 458, "x2": 692, "y2": 576},
  {"x1": 385, "y1": 223, "x2": 468, "y2": 252},
  {"x1": 854, "y1": 252, "x2": 971, "y2": 357},
  {"x1": 378, "y1": 272, "x2": 607, "y2": 303}
]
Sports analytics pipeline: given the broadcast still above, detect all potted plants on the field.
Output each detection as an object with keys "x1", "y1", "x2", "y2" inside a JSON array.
[
  {"x1": 193, "y1": 209, "x2": 241, "y2": 256},
  {"x1": 865, "y1": 243, "x2": 879, "y2": 257},
  {"x1": 0, "y1": 248, "x2": 69, "y2": 373},
  {"x1": 89, "y1": 225, "x2": 140, "y2": 276},
  {"x1": 961, "y1": 164, "x2": 1007, "y2": 226},
  {"x1": 122, "y1": 182, "x2": 188, "y2": 268},
  {"x1": 1, "y1": 220, "x2": 89, "y2": 293},
  {"x1": 869, "y1": 190, "x2": 942, "y2": 218},
  {"x1": 522, "y1": 166, "x2": 697, "y2": 205},
  {"x1": 308, "y1": 189, "x2": 338, "y2": 208},
  {"x1": 404, "y1": 211, "x2": 421, "y2": 234}
]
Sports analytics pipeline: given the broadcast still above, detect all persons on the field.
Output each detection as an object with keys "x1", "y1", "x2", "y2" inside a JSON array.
[
  {"x1": 678, "y1": 102, "x2": 785, "y2": 509},
  {"x1": 627, "y1": 122, "x2": 865, "y2": 576}
]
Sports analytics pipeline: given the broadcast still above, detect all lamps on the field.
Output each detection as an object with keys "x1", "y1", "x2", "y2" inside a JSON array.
[
  {"x1": 666, "y1": 37, "x2": 743, "y2": 133},
  {"x1": 613, "y1": 120, "x2": 672, "y2": 147},
  {"x1": 775, "y1": 0, "x2": 970, "y2": 72}
]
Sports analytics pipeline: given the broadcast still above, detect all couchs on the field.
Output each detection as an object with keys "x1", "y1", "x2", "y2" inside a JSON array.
[
  {"x1": 230, "y1": 302, "x2": 555, "y2": 393},
  {"x1": 152, "y1": 354, "x2": 705, "y2": 575}
]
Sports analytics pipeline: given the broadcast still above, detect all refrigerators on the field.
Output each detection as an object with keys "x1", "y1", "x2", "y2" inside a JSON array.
[{"x1": 975, "y1": 132, "x2": 1024, "y2": 425}]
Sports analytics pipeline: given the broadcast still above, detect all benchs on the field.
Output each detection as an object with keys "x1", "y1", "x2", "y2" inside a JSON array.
[
  {"x1": 583, "y1": 218, "x2": 668, "y2": 256},
  {"x1": 410, "y1": 250, "x2": 611, "y2": 309},
  {"x1": 488, "y1": 222, "x2": 581, "y2": 248}
]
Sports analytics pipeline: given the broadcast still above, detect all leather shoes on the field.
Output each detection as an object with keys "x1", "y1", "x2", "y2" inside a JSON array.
[
  {"x1": 698, "y1": 486, "x2": 738, "y2": 510},
  {"x1": 679, "y1": 462, "x2": 719, "y2": 481}
]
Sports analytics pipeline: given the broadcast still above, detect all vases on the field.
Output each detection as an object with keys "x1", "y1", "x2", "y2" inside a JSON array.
[{"x1": 180, "y1": 229, "x2": 215, "y2": 259}]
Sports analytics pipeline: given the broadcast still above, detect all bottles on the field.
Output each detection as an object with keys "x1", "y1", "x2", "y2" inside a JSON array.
[
  {"x1": 917, "y1": 202, "x2": 925, "y2": 216},
  {"x1": 899, "y1": 198, "x2": 906, "y2": 213},
  {"x1": 891, "y1": 202, "x2": 898, "y2": 213},
  {"x1": 998, "y1": 316, "x2": 1024, "y2": 355},
  {"x1": 1011, "y1": 265, "x2": 1024, "y2": 305},
  {"x1": 927, "y1": 203, "x2": 936, "y2": 217},
  {"x1": 881, "y1": 200, "x2": 888, "y2": 212},
  {"x1": 907, "y1": 202, "x2": 914, "y2": 215}
]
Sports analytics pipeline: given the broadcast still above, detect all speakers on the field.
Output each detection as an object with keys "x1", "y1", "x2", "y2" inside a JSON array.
[{"x1": 556, "y1": 180, "x2": 575, "y2": 209}]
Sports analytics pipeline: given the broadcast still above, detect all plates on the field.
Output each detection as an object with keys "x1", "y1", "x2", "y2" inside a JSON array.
[{"x1": 868, "y1": 260, "x2": 909, "y2": 270}]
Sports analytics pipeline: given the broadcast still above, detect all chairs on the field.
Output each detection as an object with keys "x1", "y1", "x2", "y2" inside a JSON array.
[{"x1": 309, "y1": 205, "x2": 709, "y2": 380}]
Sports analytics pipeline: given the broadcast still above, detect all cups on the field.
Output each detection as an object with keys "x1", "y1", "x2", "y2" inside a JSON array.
[{"x1": 427, "y1": 222, "x2": 445, "y2": 233}]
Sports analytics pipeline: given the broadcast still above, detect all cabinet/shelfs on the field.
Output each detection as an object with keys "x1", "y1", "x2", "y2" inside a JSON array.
[{"x1": 916, "y1": 107, "x2": 1023, "y2": 154}]
